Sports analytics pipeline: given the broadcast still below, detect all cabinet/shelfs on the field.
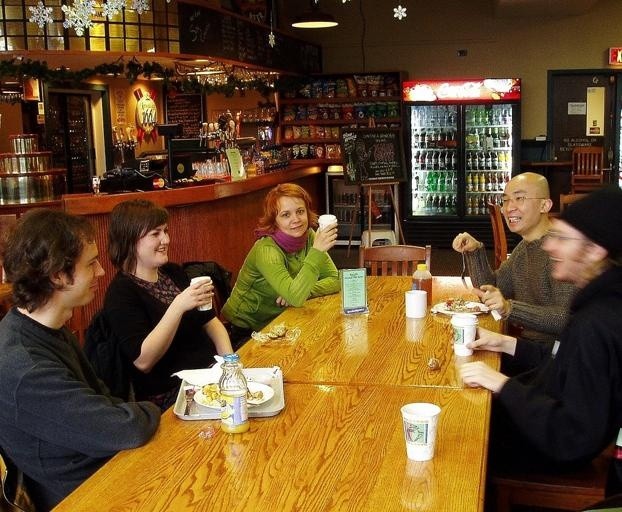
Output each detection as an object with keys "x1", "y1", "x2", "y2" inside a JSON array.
[{"x1": 271, "y1": 71, "x2": 409, "y2": 163}]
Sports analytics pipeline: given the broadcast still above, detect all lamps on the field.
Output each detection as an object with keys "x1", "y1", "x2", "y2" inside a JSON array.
[{"x1": 292, "y1": 1, "x2": 341, "y2": 30}]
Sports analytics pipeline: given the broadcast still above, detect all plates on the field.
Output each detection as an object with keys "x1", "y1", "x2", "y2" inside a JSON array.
[
  {"x1": 434, "y1": 302, "x2": 487, "y2": 316},
  {"x1": 193, "y1": 381, "x2": 274, "y2": 409}
]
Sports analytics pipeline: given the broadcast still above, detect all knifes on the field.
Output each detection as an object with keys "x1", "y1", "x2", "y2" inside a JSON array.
[{"x1": 184, "y1": 384, "x2": 195, "y2": 417}]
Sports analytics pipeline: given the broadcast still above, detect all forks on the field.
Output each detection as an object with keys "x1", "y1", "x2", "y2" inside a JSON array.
[{"x1": 460, "y1": 251, "x2": 469, "y2": 290}]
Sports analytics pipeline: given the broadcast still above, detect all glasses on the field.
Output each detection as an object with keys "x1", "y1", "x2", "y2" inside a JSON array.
[{"x1": 499, "y1": 196, "x2": 547, "y2": 208}]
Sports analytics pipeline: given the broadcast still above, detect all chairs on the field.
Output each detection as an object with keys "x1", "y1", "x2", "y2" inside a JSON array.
[
  {"x1": 570, "y1": 145, "x2": 605, "y2": 192},
  {"x1": 86, "y1": 253, "x2": 251, "y2": 409},
  {"x1": 0, "y1": 445, "x2": 47, "y2": 512},
  {"x1": 486, "y1": 202, "x2": 516, "y2": 268},
  {"x1": 560, "y1": 193, "x2": 591, "y2": 214},
  {"x1": 356, "y1": 241, "x2": 433, "y2": 275}
]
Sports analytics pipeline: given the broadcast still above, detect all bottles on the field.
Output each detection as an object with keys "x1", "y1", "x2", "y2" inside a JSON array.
[
  {"x1": 200, "y1": 122, "x2": 224, "y2": 150},
  {"x1": 412, "y1": 102, "x2": 510, "y2": 215},
  {"x1": 371, "y1": 190, "x2": 392, "y2": 205},
  {"x1": 413, "y1": 264, "x2": 433, "y2": 305},
  {"x1": 219, "y1": 355, "x2": 250, "y2": 432},
  {"x1": 47, "y1": 94, "x2": 92, "y2": 195}
]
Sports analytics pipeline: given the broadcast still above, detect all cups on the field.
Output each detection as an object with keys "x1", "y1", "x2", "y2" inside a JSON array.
[
  {"x1": 318, "y1": 214, "x2": 337, "y2": 243},
  {"x1": 451, "y1": 313, "x2": 479, "y2": 357},
  {"x1": 405, "y1": 319, "x2": 424, "y2": 342},
  {"x1": 2, "y1": 133, "x2": 68, "y2": 200},
  {"x1": 401, "y1": 402, "x2": 441, "y2": 461},
  {"x1": 191, "y1": 159, "x2": 228, "y2": 179},
  {"x1": 189, "y1": 276, "x2": 213, "y2": 310},
  {"x1": 405, "y1": 290, "x2": 428, "y2": 317}
]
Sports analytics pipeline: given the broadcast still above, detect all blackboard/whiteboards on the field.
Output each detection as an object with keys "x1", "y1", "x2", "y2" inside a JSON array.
[
  {"x1": 176, "y1": 0, "x2": 325, "y2": 78},
  {"x1": 339, "y1": 127, "x2": 409, "y2": 185}
]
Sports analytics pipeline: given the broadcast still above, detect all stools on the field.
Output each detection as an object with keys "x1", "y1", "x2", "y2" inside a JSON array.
[
  {"x1": 362, "y1": 227, "x2": 399, "y2": 251},
  {"x1": 490, "y1": 456, "x2": 614, "y2": 510}
]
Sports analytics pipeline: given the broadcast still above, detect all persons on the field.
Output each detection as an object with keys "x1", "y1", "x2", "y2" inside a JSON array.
[
  {"x1": 220, "y1": 183, "x2": 340, "y2": 351},
  {"x1": 0, "y1": 209, "x2": 161, "y2": 512},
  {"x1": 452, "y1": 172, "x2": 581, "y2": 377},
  {"x1": 458, "y1": 187, "x2": 622, "y2": 479},
  {"x1": 103, "y1": 199, "x2": 234, "y2": 413}
]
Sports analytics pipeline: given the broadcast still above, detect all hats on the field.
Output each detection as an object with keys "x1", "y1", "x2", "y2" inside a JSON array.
[{"x1": 556, "y1": 184, "x2": 621, "y2": 262}]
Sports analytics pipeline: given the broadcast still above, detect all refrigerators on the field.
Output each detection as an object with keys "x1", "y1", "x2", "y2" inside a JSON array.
[{"x1": 399, "y1": 77, "x2": 521, "y2": 252}]
